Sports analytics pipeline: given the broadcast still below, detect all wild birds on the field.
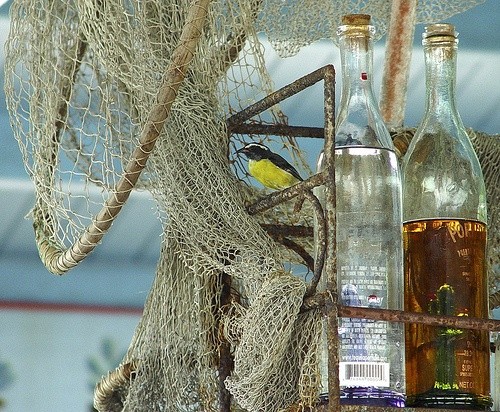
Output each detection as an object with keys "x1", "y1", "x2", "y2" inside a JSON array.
[{"x1": 233, "y1": 142, "x2": 302, "y2": 192}]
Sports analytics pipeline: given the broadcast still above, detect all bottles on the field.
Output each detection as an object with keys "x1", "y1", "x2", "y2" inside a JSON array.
[
  {"x1": 402, "y1": 23, "x2": 494, "y2": 408},
  {"x1": 310, "y1": 14, "x2": 406, "y2": 409}
]
